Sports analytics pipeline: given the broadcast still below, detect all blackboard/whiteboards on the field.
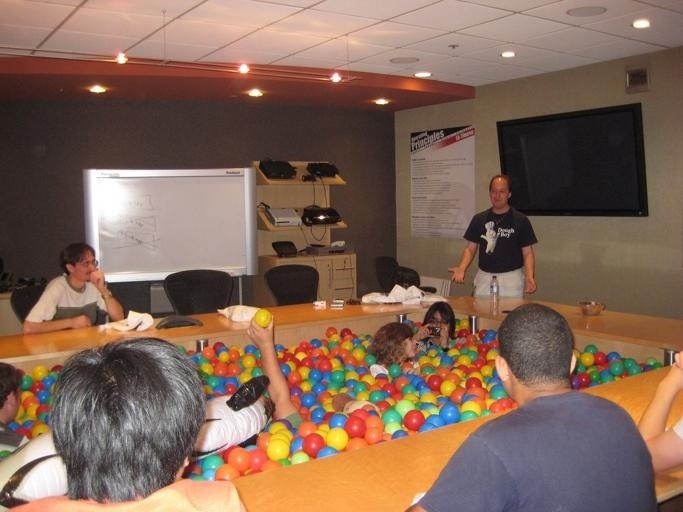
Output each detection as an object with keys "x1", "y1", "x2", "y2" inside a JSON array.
[{"x1": 82, "y1": 167, "x2": 260, "y2": 284}]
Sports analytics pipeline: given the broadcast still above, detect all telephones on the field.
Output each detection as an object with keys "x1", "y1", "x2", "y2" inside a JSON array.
[{"x1": 155, "y1": 315, "x2": 202, "y2": 329}]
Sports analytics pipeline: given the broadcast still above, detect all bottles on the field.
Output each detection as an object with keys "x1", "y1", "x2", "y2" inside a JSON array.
[{"x1": 489, "y1": 275, "x2": 499, "y2": 307}]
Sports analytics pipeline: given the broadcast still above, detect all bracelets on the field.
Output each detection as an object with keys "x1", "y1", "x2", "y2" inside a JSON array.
[{"x1": 102, "y1": 291, "x2": 113, "y2": 300}]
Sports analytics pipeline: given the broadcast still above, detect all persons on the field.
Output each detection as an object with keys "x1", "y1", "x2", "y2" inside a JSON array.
[
  {"x1": 637, "y1": 350, "x2": 682, "y2": 476},
  {"x1": 246, "y1": 316, "x2": 302, "y2": 428},
  {"x1": 367, "y1": 320, "x2": 422, "y2": 378},
  {"x1": 1, "y1": 362, "x2": 29, "y2": 457},
  {"x1": 413, "y1": 302, "x2": 457, "y2": 352},
  {"x1": 21, "y1": 242, "x2": 124, "y2": 335},
  {"x1": 446, "y1": 173, "x2": 537, "y2": 301},
  {"x1": 403, "y1": 301, "x2": 658, "y2": 512},
  {"x1": 6, "y1": 334, "x2": 243, "y2": 512}
]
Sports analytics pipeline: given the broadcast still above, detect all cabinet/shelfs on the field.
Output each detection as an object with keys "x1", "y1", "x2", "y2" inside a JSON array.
[{"x1": 250, "y1": 159, "x2": 356, "y2": 307}]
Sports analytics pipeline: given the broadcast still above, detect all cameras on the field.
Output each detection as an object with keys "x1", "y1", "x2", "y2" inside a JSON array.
[{"x1": 427, "y1": 327, "x2": 441, "y2": 337}]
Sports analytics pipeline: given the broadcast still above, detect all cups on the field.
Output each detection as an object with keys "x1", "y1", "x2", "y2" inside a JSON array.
[{"x1": 577, "y1": 300, "x2": 606, "y2": 316}]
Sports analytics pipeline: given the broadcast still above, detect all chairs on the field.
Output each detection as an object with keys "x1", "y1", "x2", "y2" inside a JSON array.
[
  {"x1": 8, "y1": 283, "x2": 46, "y2": 321},
  {"x1": 164, "y1": 270, "x2": 234, "y2": 315},
  {"x1": 262, "y1": 263, "x2": 319, "y2": 307}
]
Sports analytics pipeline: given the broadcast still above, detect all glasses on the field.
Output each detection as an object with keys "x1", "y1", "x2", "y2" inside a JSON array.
[
  {"x1": 75, "y1": 260, "x2": 98, "y2": 268},
  {"x1": 428, "y1": 319, "x2": 451, "y2": 326}
]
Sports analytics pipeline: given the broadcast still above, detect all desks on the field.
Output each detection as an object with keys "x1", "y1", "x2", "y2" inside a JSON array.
[{"x1": 0, "y1": 292, "x2": 682, "y2": 511}]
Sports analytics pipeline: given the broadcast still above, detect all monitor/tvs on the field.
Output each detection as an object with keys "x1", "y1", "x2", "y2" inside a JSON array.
[{"x1": 496, "y1": 103, "x2": 648, "y2": 217}]
